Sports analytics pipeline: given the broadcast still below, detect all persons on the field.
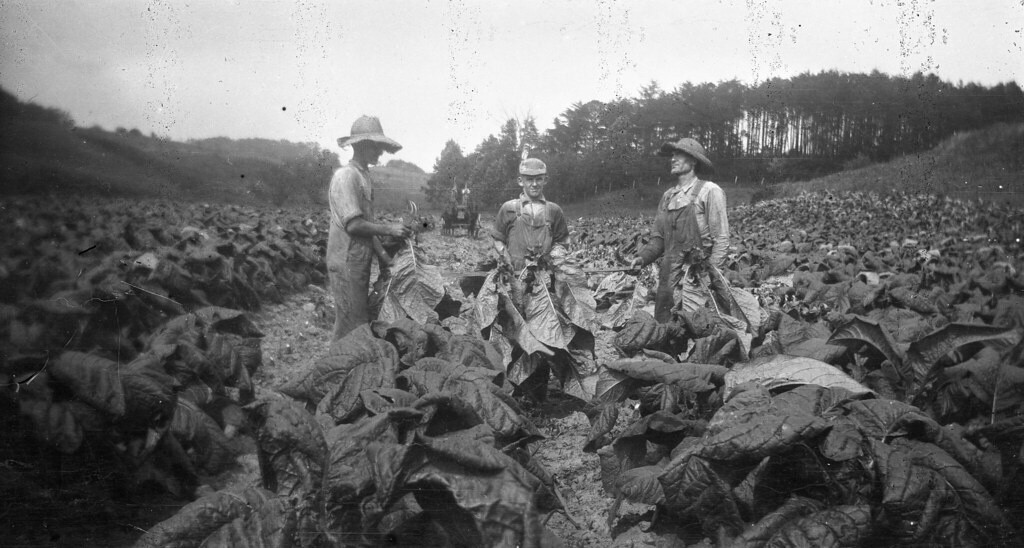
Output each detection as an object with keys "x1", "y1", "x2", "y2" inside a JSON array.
[
  {"x1": 326, "y1": 114, "x2": 412, "y2": 335},
  {"x1": 628, "y1": 137, "x2": 730, "y2": 324},
  {"x1": 491, "y1": 158, "x2": 569, "y2": 270}
]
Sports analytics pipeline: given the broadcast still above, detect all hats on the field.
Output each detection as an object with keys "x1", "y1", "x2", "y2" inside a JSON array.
[
  {"x1": 519, "y1": 158, "x2": 547, "y2": 175},
  {"x1": 336, "y1": 115, "x2": 403, "y2": 154},
  {"x1": 660, "y1": 137, "x2": 714, "y2": 175}
]
